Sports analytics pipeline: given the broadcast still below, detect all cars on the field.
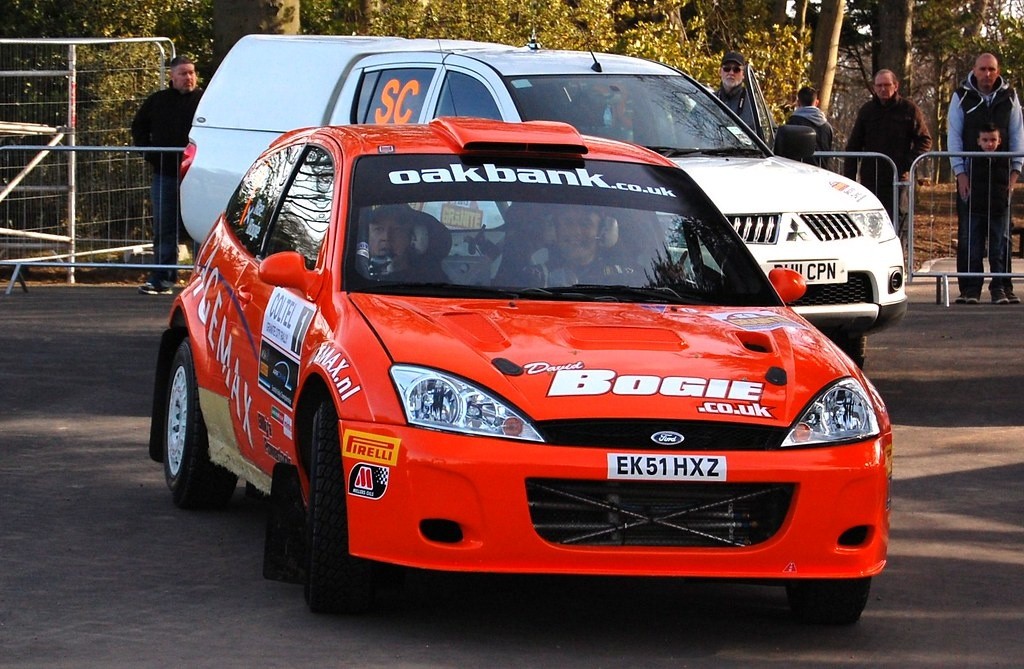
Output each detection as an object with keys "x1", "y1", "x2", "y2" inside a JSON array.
[{"x1": 146, "y1": 117, "x2": 895, "y2": 626}]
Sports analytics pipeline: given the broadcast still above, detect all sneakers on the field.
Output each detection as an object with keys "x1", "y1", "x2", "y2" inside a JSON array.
[{"x1": 138, "y1": 282, "x2": 173, "y2": 295}]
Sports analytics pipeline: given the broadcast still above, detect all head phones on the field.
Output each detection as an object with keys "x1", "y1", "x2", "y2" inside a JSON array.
[
  {"x1": 540, "y1": 211, "x2": 619, "y2": 251},
  {"x1": 357, "y1": 223, "x2": 431, "y2": 261}
]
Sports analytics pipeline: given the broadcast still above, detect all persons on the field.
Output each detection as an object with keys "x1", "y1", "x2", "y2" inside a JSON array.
[
  {"x1": 945, "y1": 53, "x2": 1024, "y2": 303},
  {"x1": 520, "y1": 204, "x2": 655, "y2": 289},
  {"x1": 957, "y1": 122, "x2": 1012, "y2": 304},
  {"x1": 688, "y1": 51, "x2": 760, "y2": 150},
  {"x1": 843, "y1": 69, "x2": 931, "y2": 243},
  {"x1": 353, "y1": 201, "x2": 454, "y2": 285},
  {"x1": 785, "y1": 87, "x2": 833, "y2": 171},
  {"x1": 131, "y1": 56, "x2": 204, "y2": 295}
]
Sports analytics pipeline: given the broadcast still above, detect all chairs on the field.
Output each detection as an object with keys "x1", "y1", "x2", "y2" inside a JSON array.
[
  {"x1": 491, "y1": 201, "x2": 557, "y2": 288},
  {"x1": 363, "y1": 210, "x2": 453, "y2": 283}
]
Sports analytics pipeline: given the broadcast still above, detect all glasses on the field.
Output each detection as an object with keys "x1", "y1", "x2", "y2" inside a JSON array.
[{"x1": 722, "y1": 66, "x2": 743, "y2": 74}]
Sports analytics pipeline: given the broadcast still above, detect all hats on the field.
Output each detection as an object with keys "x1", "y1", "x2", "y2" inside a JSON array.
[{"x1": 722, "y1": 52, "x2": 744, "y2": 65}]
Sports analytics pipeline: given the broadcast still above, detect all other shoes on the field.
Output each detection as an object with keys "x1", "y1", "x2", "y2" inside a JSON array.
[
  {"x1": 1006, "y1": 292, "x2": 1021, "y2": 302},
  {"x1": 967, "y1": 297, "x2": 979, "y2": 304},
  {"x1": 990, "y1": 289, "x2": 1009, "y2": 303},
  {"x1": 955, "y1": 292, "x2": 967, "y2": 302}
]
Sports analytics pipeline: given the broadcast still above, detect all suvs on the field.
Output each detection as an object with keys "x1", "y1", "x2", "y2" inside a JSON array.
[{"x1": 176, "y1": 31, "x2": 908, "y2": 379}]
